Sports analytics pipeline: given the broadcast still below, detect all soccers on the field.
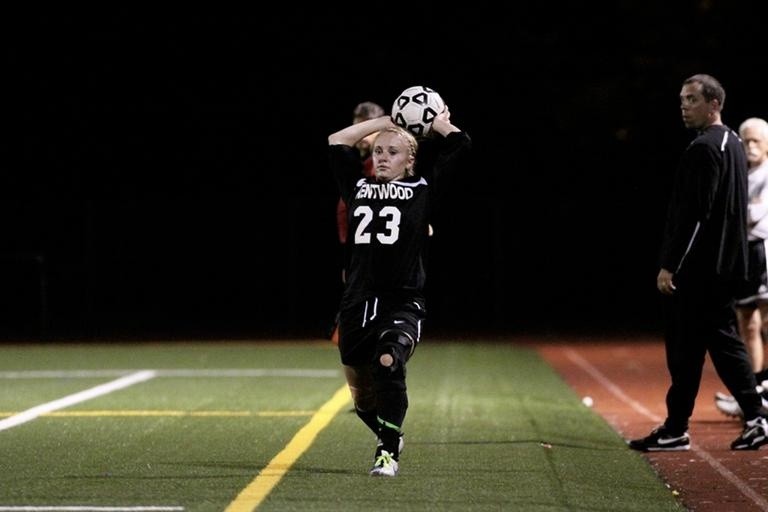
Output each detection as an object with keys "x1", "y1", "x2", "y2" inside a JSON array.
[{"x1": 392, "y1": 86, "x2": 445, "y2": 137}]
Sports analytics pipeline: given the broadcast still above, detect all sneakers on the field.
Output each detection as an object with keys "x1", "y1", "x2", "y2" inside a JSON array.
[
  {"x1": 629, "y1": 425, "x2": 690, "y2": 451},
  {"x1": 370, "y1": 436, "x2": 404, "y2": 477},
  {"x1": 715, "y1": 380, "x2": 768, "y2": 450}
]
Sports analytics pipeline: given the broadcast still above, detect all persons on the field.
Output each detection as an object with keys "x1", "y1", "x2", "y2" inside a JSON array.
[
  {"x1": 712, "y1": 118, "x2": 768, "y2": 416},
  {"x1": 328, "y1": 104, "x2": 473, "y2": 477},
  {"x1": 329, "y1": 101, "x2": 385, "y2": 405},
  {"x1": 626, "y1": 73, "x2": 768, "y2": 452}
]
